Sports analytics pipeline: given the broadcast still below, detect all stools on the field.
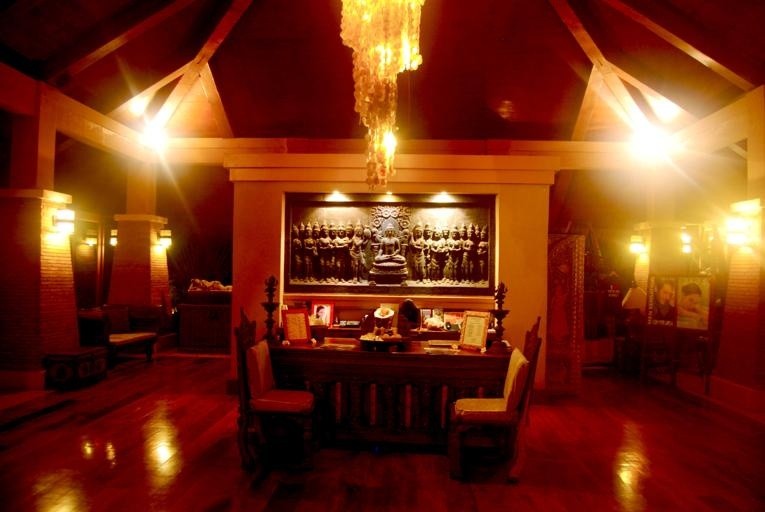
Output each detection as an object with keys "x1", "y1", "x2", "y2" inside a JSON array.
[{"x1": 45, "y1": 346, "x2": 110, "y2": 387}]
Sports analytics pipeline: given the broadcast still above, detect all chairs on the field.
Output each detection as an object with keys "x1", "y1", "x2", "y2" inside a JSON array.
[
  {"x1": 80, "y1": 310, "x2": 160, "y2": 365},
  {"x1": 232, "y1": 305, "x2": 318, "y2": 473},
  {"x1": 102, "y1": 302, "x2": 130, "y2": 330},
  {"x1": 446, "y1": 315, "x2": 543, "y2": 485}
]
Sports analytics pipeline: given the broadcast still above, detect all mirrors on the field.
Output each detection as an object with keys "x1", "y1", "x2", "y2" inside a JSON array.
[{"x1": 71, "y1": 215, "x2": 102, "y2": 307}]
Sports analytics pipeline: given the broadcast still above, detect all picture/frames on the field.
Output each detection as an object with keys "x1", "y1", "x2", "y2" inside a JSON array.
[
  {"x1": 460, "y1": 310, "x2": 491, "y2": 352},
  {"x1": 310, "y1": 298, "x2": 335, "y2": 327},
  {"x1": 283, "y1": 307, "x2": 310, "y2": 345}
]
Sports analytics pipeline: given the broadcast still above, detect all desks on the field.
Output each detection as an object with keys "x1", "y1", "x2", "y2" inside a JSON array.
[{"x1": 258, "y1": 332, "x2": 513, "y2": 456}]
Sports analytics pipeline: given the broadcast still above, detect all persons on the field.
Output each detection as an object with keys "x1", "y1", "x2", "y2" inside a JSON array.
[
  {"x1": 291, "y1": 220, "x2": 488, "y2": 284},
  {"x1": 677, "y1": 282, "x2": 709, "y2": 323},
  {"x1": 652, "y1": 278, "x2": 676, "y2": 323},
  {"x1": 316, "y1": 306, "x2": 326, "y2": 321}
]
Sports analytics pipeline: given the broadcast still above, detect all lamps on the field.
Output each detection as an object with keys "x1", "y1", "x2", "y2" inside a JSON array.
[
  {"x1": 335, "y1": 2, "x2": 428, "y2": 194},
  {"x1": 622, "y1": 254, "x2": 648, "y2": 310}
]
